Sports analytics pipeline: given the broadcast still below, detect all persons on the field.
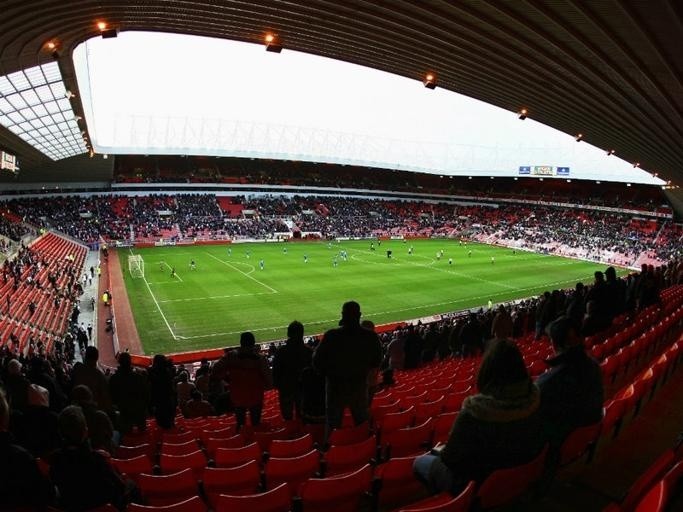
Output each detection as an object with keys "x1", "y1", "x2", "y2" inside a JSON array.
[
  {"x1": 0, "y1": 166, "x2": 682, "y2": 317},
  {"x1": 1, "y1": 270, "x2": 618, "y2": 510}
]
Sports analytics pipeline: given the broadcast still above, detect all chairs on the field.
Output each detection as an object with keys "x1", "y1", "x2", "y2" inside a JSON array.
[
  {"x1": 105, "y1": 284, "x2": 682, "y2": 510},
  {"x1": 0, "y1": 206, "x2": 89, "y2": 372},
  {"x1": 441, "y1": 191, "x2": 682, "y2": 268},
  {"x1": 94, "y1": 195, "x2": 439, "y2": 243},
  {"x1": 115, "y1": 154, "x2": 439, "y2": 195}
]
